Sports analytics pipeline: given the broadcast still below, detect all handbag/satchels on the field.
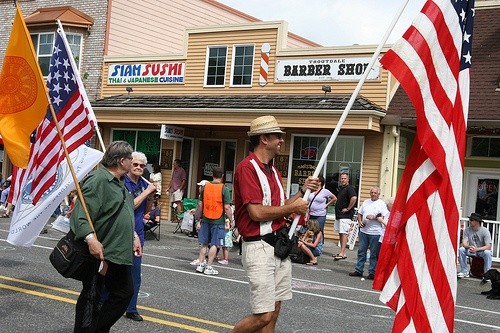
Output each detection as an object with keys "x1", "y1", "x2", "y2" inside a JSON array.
[
  {"x1": 49, "y1": 231, "x2": 93, "y2": 280},
  {"x1": 272, "y1": 230, "x2": 295, "y2": 259},
  {"x1": 181, "y1": 212, "x2": 194, "y2": 232},
  {"x1": 225, "y1": 229, "x2": 233, "y2": 248}
]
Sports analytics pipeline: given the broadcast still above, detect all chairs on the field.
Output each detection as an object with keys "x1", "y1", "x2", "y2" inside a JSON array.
[
  {"x1": 173, "y1": 198, "x2": 199, "y2": 234},
  {"x1": 143, "y1": 210, "x2": 160, "y2": 241}
]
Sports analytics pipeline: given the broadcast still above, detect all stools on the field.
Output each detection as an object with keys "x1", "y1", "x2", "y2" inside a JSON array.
[{"x1": 467, "y1": 255, "x2": 484, "y2": 279}]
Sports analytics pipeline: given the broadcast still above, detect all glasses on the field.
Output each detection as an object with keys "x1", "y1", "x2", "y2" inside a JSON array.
[{"x1": 132, "y1": 163, "x2": 145, "y2": 168}]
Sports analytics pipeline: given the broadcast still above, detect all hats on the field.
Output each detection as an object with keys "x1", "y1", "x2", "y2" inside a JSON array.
[
  {"x1": 247, "y1": 116, "x2": 286, "y2": 136},
  {"x1": 197, "y1": 180, "x2": 208, "y2": 186},
  {"x1": 467, "y1": 213, "x2": 484, "y2": 223}
]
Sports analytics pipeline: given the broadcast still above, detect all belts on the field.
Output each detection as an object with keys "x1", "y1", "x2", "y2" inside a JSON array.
[{"x1": 243, "y1": 235, "x2": 262, "y2": 242}]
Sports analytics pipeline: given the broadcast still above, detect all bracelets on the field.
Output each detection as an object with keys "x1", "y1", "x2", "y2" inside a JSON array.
[
  {"x1": 300, "y1": 188, "x2": 305, "y2": 195},
  {"x1": 86, "y1": 234, "x2": 93, "y2": 242}
]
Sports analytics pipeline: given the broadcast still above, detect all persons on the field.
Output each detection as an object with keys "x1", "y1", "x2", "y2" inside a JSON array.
[
  {"x1": 229, "y1": 116, "x2": 320, "y2": 333},
  {"x1": 165, "y1": 159, "x2": 186, "y2": 223},
  {"x1": 333, "y1": 172, "x2": 357, "y2": 259},
  {"x1": 308, "y1": 175, "x2": 337, "y2": 244},
  {"x1": 196, "y1": 167, "x2": 234, "y2": 275},
  {"x1": 457, "y1": 213, "x2": 492, "y2": 282},
  {"x1": 298, "y1": 219, "x2": 324, "y2": 265},
  {"x1": 376, "y1": 197, "x2": 395, "y2": 261},
  {"x1": 189, "y1": 180, "x2": 208, "y2": 266},
  {"x1": 206, "y1": 244, "x2": 220, "y2": 260},
  {"x1": 124, "y1": 152, "x2": 157, "y2": 321},
  {"x1": 70, "y1": 141, "x2": 142, "y2": 333},
  {"x1": 349, "y1": 186, "x2": 389, "y2": 280},
  {"x1": 0, "y1": 163, "x2": 162, "y2": 238},
  {"x1": 218, "y1": 204, "x2": 233, "y2": 264}
]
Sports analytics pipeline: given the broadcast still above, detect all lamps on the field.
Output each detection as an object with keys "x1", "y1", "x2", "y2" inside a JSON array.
[
  {"x1": 126, "y1": 87, "x2": 133, "y2": 95},
  {"x1": 322, "y1": 84, "x2": 331, "y2": 95}
]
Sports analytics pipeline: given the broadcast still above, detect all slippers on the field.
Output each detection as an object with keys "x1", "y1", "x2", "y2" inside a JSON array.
[
  {"x1": 306, "y1": 257, "x2": 318, "y2": 265},
  {"x1": 333, "y1": 253, "x2": 347, "y2": 259}
]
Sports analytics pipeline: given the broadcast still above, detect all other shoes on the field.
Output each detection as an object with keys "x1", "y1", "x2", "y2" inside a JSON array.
[
  {"x1": 367, "y1": 274, "x2": 374, "y2": 279},
  {"x1": 204, "y1": 266, "x2": 218, "y2": 274},
  {"x1": 218, "y1": 259, "x2": 228, "y2": 264},
  {"x1": 126, "y1": 312, "x2": 142, "y2": 321},
  {"x1": 196, "y1": 263, "x2": 206, "y2": 272},
  {"x1": 458, "y1": 272, "x2": 469, "y2": 278},
  {"x1": 349, "y1": 272, "x2": 362, "y2": 276},
  {"x1": 189, "y1": 234, "x2": 198, "y2": 237},
  {"x1": 190, "y1": 259, "x2": 206, "y2": 265}
]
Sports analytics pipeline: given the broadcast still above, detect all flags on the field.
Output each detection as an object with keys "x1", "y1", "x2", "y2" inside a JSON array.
[
  {"x1": 372, "y1": 0, "x2": 475, "y2": 333},
  {"x1": 6, "y1": 143, "x2": 104, "y2": 248},
  {"x1": 0, "y1": 8, "x2": 49, "y2": 169},
  {"x1": 7, "y1": 28, "x2": 96, "y2": 206}
]
